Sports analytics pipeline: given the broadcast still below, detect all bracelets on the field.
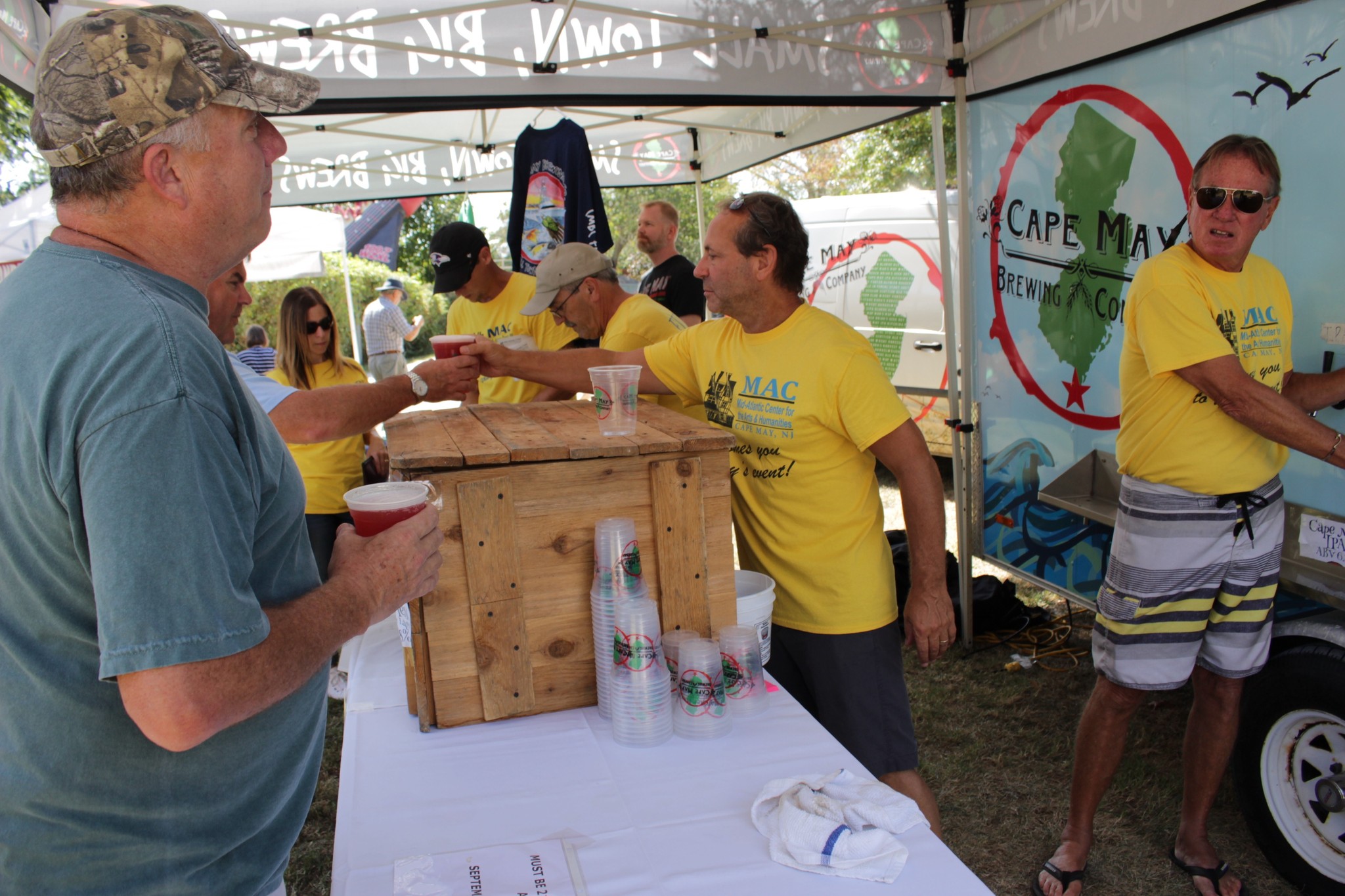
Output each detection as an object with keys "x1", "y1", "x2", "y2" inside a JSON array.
[{"x1": 1322, "y1": 430, "x2": 1341, "y2": 461}]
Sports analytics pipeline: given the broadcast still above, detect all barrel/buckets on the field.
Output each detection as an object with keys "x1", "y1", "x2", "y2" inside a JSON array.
[{"x1": 735, "y1": 570, "x2": 776, "y2": 666}]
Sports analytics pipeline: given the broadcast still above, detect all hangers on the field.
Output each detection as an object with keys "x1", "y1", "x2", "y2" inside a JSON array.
[{"x1": 532, "y1": 106, "x2": 564, "y2": 127}]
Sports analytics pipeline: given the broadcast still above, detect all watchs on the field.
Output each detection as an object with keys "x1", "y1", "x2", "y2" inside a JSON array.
[{"x1": 405, "y1": 371, "x2": 428, "y2": 404}]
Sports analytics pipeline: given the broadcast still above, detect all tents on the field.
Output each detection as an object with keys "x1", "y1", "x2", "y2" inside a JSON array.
[
  {"x1": 0, "y1": 180, "x2": 360, "y2": 364},
  {"x1": 0, "y1": 0, "x2": 1299, "y2": 634}
]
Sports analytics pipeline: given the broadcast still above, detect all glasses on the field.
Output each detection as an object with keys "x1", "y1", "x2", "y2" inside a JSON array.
[
  {"x1": 305, "y1": 317, "x2": 334, "y2": 335},
  {"x1": 550, "y1": 276, "x2": 597, "y2": 318},
  {"x1": 729, "y1": 198, "x2": 773, "y2": 245},
  {"x1": 1195, "y1": 186, "x2": 1275, "y2": 214}
]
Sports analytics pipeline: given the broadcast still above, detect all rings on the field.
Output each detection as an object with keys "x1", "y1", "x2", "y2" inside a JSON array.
[
  {"x1": 384, "y1": 459, "x2": 388, "y2": 462},
  {"x1": 940, "y1": 639, "x2": 949, "y2": 643}
]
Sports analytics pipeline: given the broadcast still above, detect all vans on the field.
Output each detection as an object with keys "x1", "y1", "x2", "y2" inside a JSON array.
[{"x1": 790, "y1": 188, "x2": 962, "y2": 460}]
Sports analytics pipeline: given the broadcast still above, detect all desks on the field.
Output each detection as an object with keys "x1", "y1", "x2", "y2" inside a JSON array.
[{"x1": 331, "y1": 612, "x2": 993, "y2": 896}]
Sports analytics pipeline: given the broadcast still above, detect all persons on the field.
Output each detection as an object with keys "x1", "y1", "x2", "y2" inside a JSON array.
[
  {"x1": 1033, "y1": 133, "x2": 1345, "y2": 896},
  {"x1": 237, "y1": 325, "x2": 277, "y2": 375},
  {"x1": 430, "y1": 222, "x2": 579, "y2": 408},
  {"x1": 459, "y1": 193, "x2": 956, "y2": 838},
  {"x1": 265, "y1": 286, "x2": 390, "y2": 583},
  {"x1": 638, "y1": 201, "x2": 705, "y2": 328},
  {"x1": 0, "y1": 5, "x2": 442, "y2": 896},
  {"x1": 362, "y1": 279, "x2": 424, "y2": 380},
  {"x1": 521, "y1": 243, "x2": 708, "y2": 422},
  {"x1": 207, "y1": 252, "x2": 481, "y2": 444}
]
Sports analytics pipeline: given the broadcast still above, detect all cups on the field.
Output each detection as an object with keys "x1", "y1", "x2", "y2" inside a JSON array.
[
  {"x1": 609, "y1": 598, "x2": 673, "y2": 749},
  {"x1": 587, "y1": 365, "x2": 643, "y2": 437},
  {"x1": 343, "y1": 481, "x2": 429, "y2": 537},
  {"x1": 673, "y1": 637, "x2": 733, "y2": 741},
  {"x1": 589, "y1": 517, "x2": 649, "y2": 723},
  {"x1": 719, "y1": 624, "x2": 770, "y2": 718},
  {"x1": 428, "y1": 335, "x2": 475, "y2": 360},
  {"x1": 661, "y1": 630, "x2": 700, "y2": 713}
]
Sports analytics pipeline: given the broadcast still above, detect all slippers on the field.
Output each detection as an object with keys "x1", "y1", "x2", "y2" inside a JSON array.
[
  {"x1": 1169, "y1": 845, "x2": 1244, "y2": 896},
  {"x1": 1032, "y1": 855, "x2": 1086, "y2": 896}
]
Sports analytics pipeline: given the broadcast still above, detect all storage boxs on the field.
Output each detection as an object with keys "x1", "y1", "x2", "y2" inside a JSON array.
[{"x1": 383, "y1": 396, "x2": 738, "y2": 733}]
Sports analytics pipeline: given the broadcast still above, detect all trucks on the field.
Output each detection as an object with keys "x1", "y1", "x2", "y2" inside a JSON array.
[{"x1": 969, "y1": 0, "x2": 1345, "y2": 896}]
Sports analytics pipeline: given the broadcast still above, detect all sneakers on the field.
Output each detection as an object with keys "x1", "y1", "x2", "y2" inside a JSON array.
[{"x1": 329, "y1": 667, "x2": 347, "y2": 701}]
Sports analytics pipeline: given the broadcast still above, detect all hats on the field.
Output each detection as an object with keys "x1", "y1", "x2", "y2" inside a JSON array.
[
  {"x1": 28, "y1": 6, "x2": 322, "y2": 169},
  {"x1": 430, "y1": 222, "x2": 484, "y2": 293},
  {"x1": 520, "y1": 242, "x2": 613, "y2": 317},
  {"x1": 375, "y1": 280, "x2": 408, "y2": 301}
]
